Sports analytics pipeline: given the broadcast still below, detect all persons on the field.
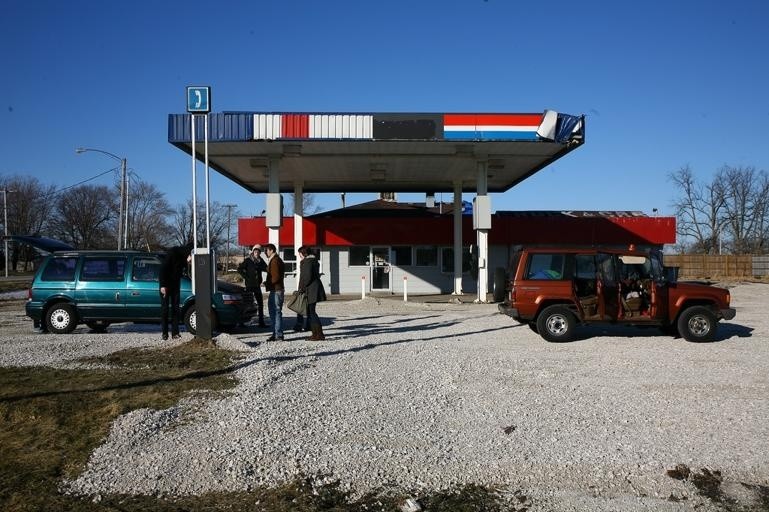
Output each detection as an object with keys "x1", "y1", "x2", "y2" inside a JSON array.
[
  {"x1": 158, "y1": 241, "x2": 195, "y2": 340},
  {"x1": 297, "y1": 245, "x2": 327, "y2": 340},
  {"x1": 33, "y1": 319, "x2": 43, "y2": 333},
  {"x1": 293, "y1": 304, "x2": 311, "y2": 331},
  {"x1": 263, "y1": 243, "x2": 286, "y2": 342},
  {"x1": 237, "y1": 244, "x2": 268, "y2": 329}
]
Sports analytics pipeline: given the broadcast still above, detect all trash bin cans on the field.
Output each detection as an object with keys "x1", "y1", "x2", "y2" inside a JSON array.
[{"x1": 663, "y1": 266, "x2": 679, "y2": 281}]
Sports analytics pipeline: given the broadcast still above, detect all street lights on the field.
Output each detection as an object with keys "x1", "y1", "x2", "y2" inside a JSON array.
[{"x1": 74, "y1": 146, "x2": 128, "y2": 250}]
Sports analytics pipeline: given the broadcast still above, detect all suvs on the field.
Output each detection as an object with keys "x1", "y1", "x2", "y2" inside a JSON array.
[
  {"x1": 25, "y1": 246, "x2": 258, "y2": 333},
  {"x1": 491, "y1": 246, "x2": 735, "y2": 342}
]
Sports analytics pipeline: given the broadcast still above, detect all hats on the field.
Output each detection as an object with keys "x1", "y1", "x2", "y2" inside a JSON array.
[{"x1": 252, "y1": 244, "x2": 261, "y2": 252}]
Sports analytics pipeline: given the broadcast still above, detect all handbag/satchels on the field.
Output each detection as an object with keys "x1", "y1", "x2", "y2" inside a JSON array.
[{"x1": 287, "y1": 291, "x2": 308, "y2": 315}]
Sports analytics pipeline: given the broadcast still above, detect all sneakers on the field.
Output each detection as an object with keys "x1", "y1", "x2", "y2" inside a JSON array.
[
  {"x1": 163, "y1": 334, "x2": 181, "y2": 340},
  {"x1": 294, "y1": 326, "x2": 311, "y2": 331},
  {"x1": 259, "y1": 324, "x2": 270, "y2": 328},
  {"x1": 267, "y1": 336, "x2": 283, "y2": 341}
]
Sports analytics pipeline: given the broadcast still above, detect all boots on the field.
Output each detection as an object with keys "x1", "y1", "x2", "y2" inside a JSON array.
[{"x1": 305, "y1": 322, "x2": 325, "y2": 341}]
